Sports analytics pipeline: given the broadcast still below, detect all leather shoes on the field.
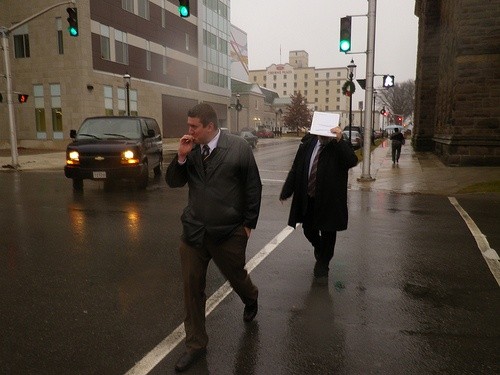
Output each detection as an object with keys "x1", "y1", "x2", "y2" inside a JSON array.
[
  {"x1": 243, "y1": 291, "x2": 258, "y2": 323},
  {"x1": 175, "y1": 348, "x2": 206, "y2": 371}
]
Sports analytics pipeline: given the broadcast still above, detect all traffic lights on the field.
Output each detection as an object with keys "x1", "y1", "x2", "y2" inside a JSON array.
[
  {"x1": 18, "y1": 94, "x2": 29, "y2": 102},
  {"x1": 66, "y1": 7, "x2": 79, "y2": 36},
  {"x1": 178, "y1": 0, "x2": 190, "y2": 18},
  {"x1": 383, "y1": 76, "x2": 395, "y2": 87},
  {"x1": 339, "y1": 17, "x2": 352, "y2": 52}
]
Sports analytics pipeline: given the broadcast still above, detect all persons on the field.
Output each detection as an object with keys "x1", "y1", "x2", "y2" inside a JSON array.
[
  {"x1": 390, "y1": 128, "x2": 406, "y2": 165},
  {"x1": 279, "y1": 126, "x2": 359, "y2": 276},
  {"x1": 165, "y1": 101, "x2": 263, "y2": 372}
]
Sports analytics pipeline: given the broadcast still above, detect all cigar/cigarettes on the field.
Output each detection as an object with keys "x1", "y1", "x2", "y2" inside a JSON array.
[{"x1": 185, "y1": 140, "x2": 189, "y2": 142}]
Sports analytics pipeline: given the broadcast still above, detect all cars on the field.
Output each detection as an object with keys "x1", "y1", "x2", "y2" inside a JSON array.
[
  {"x1": 256, "y1": 128, "x2": 273, "y2": 138},
  {"x1": 235, "y1": 131, "x2": 258, "y2": 149},
  {"x1": 342, "y1": 130, "x2": 361, "y2": 147},
  {"x1": 344, "y1": 125, "x2": 384, "y2": 141}
]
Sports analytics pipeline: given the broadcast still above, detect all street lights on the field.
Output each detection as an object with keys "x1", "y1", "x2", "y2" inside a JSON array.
[
  {"x1": 346, "y1": 58, "x2": 357, "y2": 143},
  {"x1": 123, "y1": 72, "x2": 132, "y2": 116},
  {"x1": 235, "y1": 92, "x2": 240, "y2": 133}
]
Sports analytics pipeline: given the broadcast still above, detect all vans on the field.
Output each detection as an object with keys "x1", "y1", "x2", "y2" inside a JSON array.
[{"x1": 63, "y1": 116, "x2": 163, "y2": 190}]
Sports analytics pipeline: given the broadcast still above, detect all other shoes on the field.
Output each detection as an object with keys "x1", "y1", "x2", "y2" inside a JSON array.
[
  {"x1": 393, "y1": 160, "x2": 398, "y2": 163},
  {"x1": 314, "y1": 236, "x2": 329, "y2": 280}
]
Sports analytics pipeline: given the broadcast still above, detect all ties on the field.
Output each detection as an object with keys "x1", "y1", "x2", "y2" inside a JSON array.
[
  {"x1": 308, "y1": 144, "x2": 326, "y2": 198},
  {"x1": 201, "y1": 145, "x2": 210, "y2": 177}
]
